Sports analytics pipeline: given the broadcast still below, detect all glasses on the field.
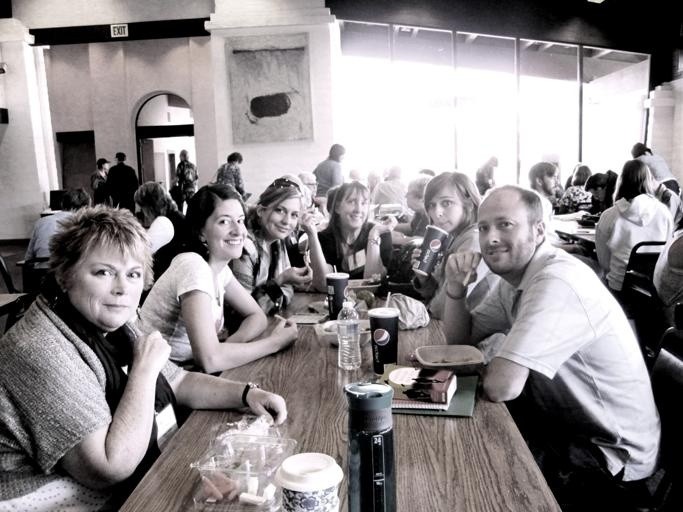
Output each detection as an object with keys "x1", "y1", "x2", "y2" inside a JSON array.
[{"x1": 270, "y1": 179, "x2": 299, "y2": 189}]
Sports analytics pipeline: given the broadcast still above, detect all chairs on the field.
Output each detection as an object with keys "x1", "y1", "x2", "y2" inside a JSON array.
[
  {"x1": 599, "y1": 322, "x2": 682, "y2": 509},
  {"x1": 624, "y1": 238, "x2": 666, "y2": 273},
  {"x1": 614, "y1": 271, "x2": 669, "y2": 368}
]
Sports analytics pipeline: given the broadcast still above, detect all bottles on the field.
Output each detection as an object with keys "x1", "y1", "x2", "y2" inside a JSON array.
[
  {"x1": 342, "y1": 380, "x2": 399, "y2": 512},
  {"x1": 336, "y1": 300, "x2": 362, "y2": 371}
]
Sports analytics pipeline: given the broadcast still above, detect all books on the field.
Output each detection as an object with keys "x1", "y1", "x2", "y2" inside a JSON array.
[{"x1": 375, "y1": 364, "x2": 458, "y2": 411}]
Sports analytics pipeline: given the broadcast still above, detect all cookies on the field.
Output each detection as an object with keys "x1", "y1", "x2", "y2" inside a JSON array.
[{"x1": 201, "y1": 441, "x2": 267, "y2": 501}]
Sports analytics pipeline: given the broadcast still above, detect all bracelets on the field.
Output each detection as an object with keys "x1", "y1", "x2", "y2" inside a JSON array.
[{"x1": 241, "y1": 381, "x2": 259, "y2": 408}]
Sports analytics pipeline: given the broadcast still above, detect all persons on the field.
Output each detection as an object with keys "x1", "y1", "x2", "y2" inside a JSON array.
[
  {"x1": 1, "y1": 203, "x2": 288, "y2": 512},
  {"x1": 444, "y1": 186, "x2": 662, "y2": 512},
  {"x1": 138, "y1": 184, "x2": 297, "y2": 373},
  {"x1": 22, "y1": 144, "x2": 683, "y2": 335}
]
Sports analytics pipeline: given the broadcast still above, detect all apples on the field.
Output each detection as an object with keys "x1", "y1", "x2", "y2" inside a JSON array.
[{"x1": 357, "y1": 290, "x2": 375, "y2": 310}]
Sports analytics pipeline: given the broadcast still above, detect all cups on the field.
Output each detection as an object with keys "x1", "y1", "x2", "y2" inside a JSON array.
[
  {"x1": 274, "y1": 452, "x2": 345, "y2": 511},
  {"x1": 411, "y1": 223, "x2": 449, "y2": 277},
  {"x1": 367, "y1": 306, "x2": 400, "y2": 378},
  {"x1": 325, "y1": 272, "x2": 351, "y2": 321}
]
